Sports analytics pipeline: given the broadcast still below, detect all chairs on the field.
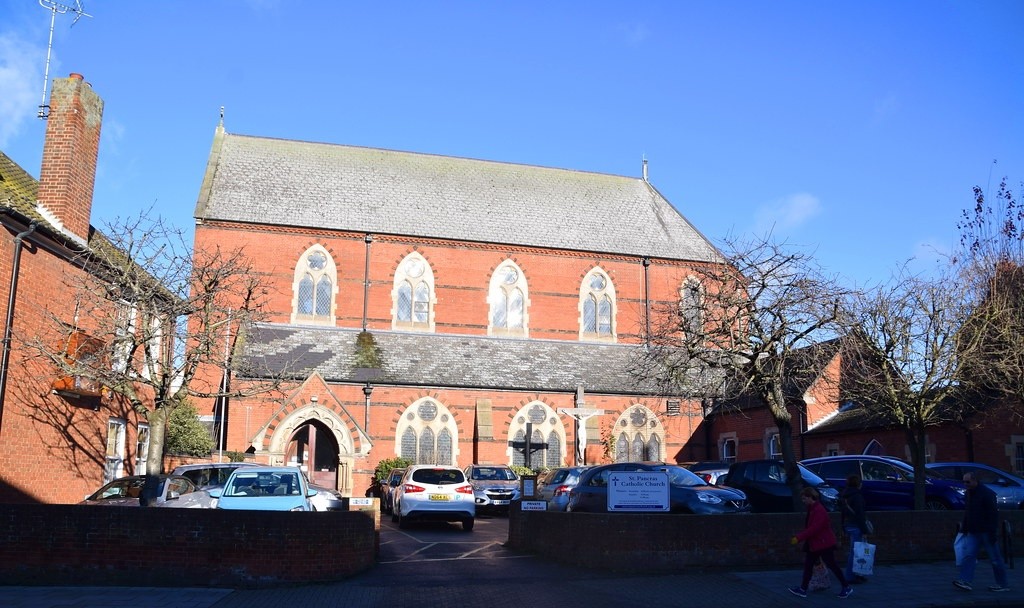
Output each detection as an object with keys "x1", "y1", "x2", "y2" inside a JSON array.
[
  {"x1": 475, "y1": 469, "x2": 480, "y2": 475},
  {"x1": 496, "y1": 471, "x2": 503, "y2": 477},
  {"x1": 280, "y1": 474, "x2": 293, "y2": 494}
]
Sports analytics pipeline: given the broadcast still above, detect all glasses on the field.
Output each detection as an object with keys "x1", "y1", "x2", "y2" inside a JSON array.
[{"x1": 961, "y1": 480, "x2": 970, "y2": 484}]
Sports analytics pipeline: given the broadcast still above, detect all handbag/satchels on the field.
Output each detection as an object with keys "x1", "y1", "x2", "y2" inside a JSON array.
[
  {"x1": 863, "y1": 520, "x2": 873, "y2": 535},
  {"x1": 954, "y1": 532, "x2": 973, "y2": 567},
  {"x1": 852, "y1": 541, "x2": 877, "y2": 577},
  {"x1": 809, "y1": 557, "x2": 831, "y2": 592}
]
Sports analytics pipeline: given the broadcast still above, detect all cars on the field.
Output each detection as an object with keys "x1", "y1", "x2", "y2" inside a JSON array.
[
  {"x1": 380, "y1": 467, "x2": 406, "y2": 516},
  {"x1": 536, "y1": 466, "x2": 590, "y2": 514},
  {"x1": 75, "y1": 473, "x2": 212, "y2": 506},
  {"x1": 678, "y1": 461, "x2": 729, "y2": 486},
  {"x1": 565, "y1": 461, "x2": 752, "y2": 516},
  {"x1": 798, "y1": 454, "x2": 1000, "y2": 512},
  {"x1": 209, "y1": 465, "x2": 318, "y2": 511},
  {"x1": 922, "y1": 462, "x2": 1024, "y2": 509},
  {"x1": 725, "y1": 458, "x2": 841, "y2": 513}
]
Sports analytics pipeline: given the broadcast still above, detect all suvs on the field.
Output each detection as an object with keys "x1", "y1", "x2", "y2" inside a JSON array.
[
  {"x1": 463, "y1": 463, "x2": 521, "y2": 517},
  {"x1": 389, "y1": 464, "x2": 475, "y2": 532},
  {"x1": 170, "y1": 464, "x2": 343, "y2": 513}
]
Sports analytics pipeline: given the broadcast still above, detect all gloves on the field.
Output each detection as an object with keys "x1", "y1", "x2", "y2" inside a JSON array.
[{"x1": 791, "y1": 537, "x2": 798, "y2": 545}]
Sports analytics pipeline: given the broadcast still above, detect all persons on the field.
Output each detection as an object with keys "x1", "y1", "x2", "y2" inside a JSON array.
[
  {"x1": 952, "y1": 472, "x2": 1011, "y2": 591},
  {"x1": 788, "y1": 489, "x2": 853, "y2": 599},
  {"x1": 839, "y1": 474, "x2": 866, "y2": 586}
]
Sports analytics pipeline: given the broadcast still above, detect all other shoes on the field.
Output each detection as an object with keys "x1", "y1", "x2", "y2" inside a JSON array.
[{"x1": 852, "y1": 577, "x2": 867, "y2": 585}]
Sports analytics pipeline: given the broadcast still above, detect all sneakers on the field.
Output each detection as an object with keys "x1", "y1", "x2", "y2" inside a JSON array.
[
  {"x1": 987, "y1": 584, "x2": 1012, "y2": 592},
  {"x1": 788, "y1": 587, "x2": 807, "y2": 598},
  {"x1": 953, "y1": 579, "x2": 973, "y2": 591},
  {"x1": 836, "y1": 586, "x2": 853, "y2": 599}
]
